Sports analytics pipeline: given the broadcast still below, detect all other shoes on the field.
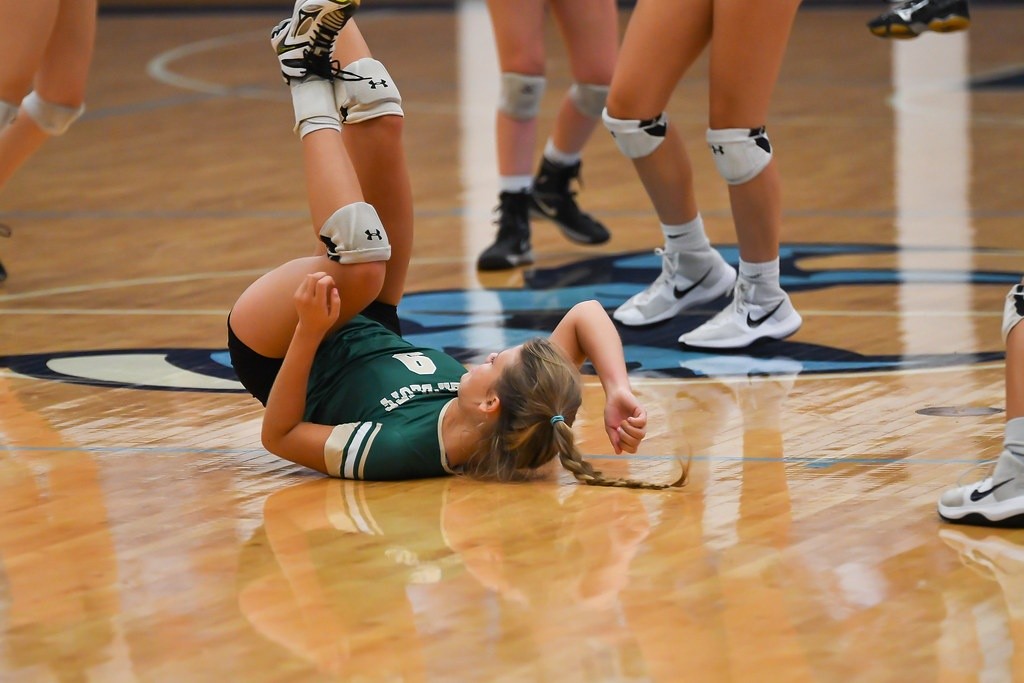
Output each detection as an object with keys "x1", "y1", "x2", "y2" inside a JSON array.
[{"x1": 0, "y1": 224, "x2": 11, "y2": 281}]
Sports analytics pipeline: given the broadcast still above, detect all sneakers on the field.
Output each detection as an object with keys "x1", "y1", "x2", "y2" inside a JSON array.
[
  {"x1": 680, "y1": 355, "x2": 803, "y2": 404},
  {"x1": 937, "y1": 452, "x2": 1024, "y2": 528},
  {"x1": 941, "y1": 531, "x2": 1024, "y2": 581},
  {"x1": 867, "y1": 0, "x2": 971, "y2": 38},
  {"x1": 678, "y1": 287, "x2": 802, "y2": 349},
  {"x1": 270, "y1": 0, "x2": 372, "y2": 85},
  {"x1": 612, "y1": 246, "x2": 737, "y2": 327}
]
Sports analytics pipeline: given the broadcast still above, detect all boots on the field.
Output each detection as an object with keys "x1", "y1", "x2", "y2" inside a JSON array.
[
  {"x1": 528, "y1": 155, "x2": 610, "y2": 246},
  {"x1": 478, "y1": 188, "x2": 531, "y2": 269}
]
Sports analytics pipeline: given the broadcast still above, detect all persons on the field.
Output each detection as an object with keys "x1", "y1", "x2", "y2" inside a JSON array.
[
  {"x1": 935, "y1": 277, "x2": 1024, "y2": 526},
  {"x1": 0, "y1": 0, "x2": 96, "y2": 279},
  {"x1": 475, "y1": 0, "x2": 624, "y2": 269},
  {"x1": 868, "y1": 0, "x2": 970, "y2": 40},
  {"x1": 592, "y1": 0, "x2": 803, "y2": 348},
  {"x1": 228, "y1": 0, "x2": 692, "y2": 491}
]
